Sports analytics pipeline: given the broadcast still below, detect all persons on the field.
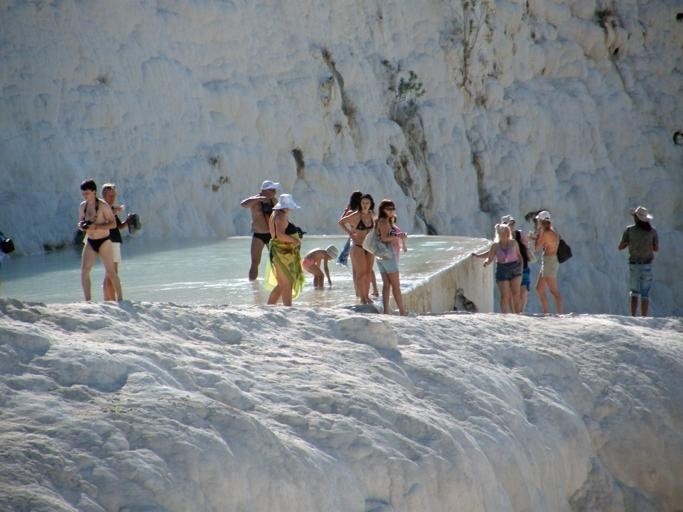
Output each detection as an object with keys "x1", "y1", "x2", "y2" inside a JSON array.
[
  {"x1": 617, "y1": 205, "x2": 659, "y2": 316},
  {"x1": 338, "y1": 191, "x2": 408, "y2": 316},
  {"x1": 263, "y1": 194, "x2": 305, "y2": 307},
  {"x1": 102, "y1": 183, "x2": 132, "y2": 301},
  {"x1": 240, "y1": 180, "x2": 280, "y2": 281},
  {"x1": 302, "y1": 244, "x2": 338, "y2": 289},
  {"x1": 472, "y1": 208, "x2": 563, "y2": 314},
  {"x1": 78, "y1": 180, "x2": 122, "y2": 301}
]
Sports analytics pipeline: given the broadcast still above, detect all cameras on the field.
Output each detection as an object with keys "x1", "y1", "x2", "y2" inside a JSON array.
[{"x1": 80, "y1": 222, "x2": 89, "y2": 230}]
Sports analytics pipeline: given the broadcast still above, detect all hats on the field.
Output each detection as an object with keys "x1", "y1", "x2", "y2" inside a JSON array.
[
  {"x1": 629, "y1": 206, "x2": 654, "y2": 222},
  {"x1": 261, "y1": 180, "x2": 280, "y2": 190},
  {"x1": 500, "y1": 215, "x2": 516, "y2": 224},
  {"x1": 326, "y1": 246, "x2": 338, "y2": 259},
  {"x1": 535, "y1": 211, "x2": 551, "y2": 222},
  {"x1": 271, "y1": 193, "x2": 301, "y2": 210}
]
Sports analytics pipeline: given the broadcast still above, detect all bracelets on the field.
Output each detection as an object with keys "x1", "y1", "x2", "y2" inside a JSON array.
[{"x1": 95, "y1": 224, "x2": 97, "y2": 229}]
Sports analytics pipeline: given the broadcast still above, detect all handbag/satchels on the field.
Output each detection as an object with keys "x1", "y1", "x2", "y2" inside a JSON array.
[
  {"x1": 362, "y1": 229, "x2": 393, "y2": 260},
  {"x1": 557, "y1": 239, "x2": 572, "y2": 263}
]
